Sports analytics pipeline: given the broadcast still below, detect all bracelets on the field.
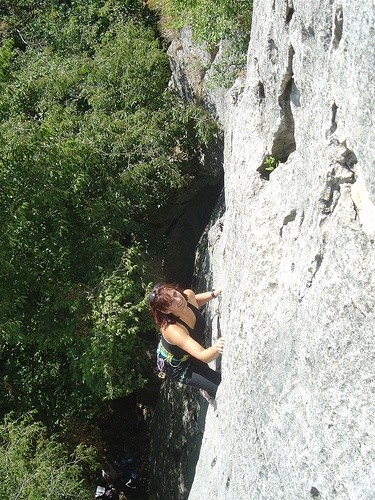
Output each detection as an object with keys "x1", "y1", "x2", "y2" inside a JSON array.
[{"x1": 210, "y1": 290, "x2": 216, "y2": 299}]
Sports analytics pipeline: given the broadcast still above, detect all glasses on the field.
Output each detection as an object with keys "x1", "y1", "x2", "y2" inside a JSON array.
[{"x1": 150, "y1": 285, "x2": 160, "y2": 301}]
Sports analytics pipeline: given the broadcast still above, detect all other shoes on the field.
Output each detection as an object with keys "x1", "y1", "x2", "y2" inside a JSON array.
[{"x1": 201, "y1": 389, "x2": 213, "y2": 404}]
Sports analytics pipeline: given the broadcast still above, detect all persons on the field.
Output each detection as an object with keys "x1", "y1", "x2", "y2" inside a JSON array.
[{"x1": 148, "y1": 283, "x2": 224, "y2": 407}]
